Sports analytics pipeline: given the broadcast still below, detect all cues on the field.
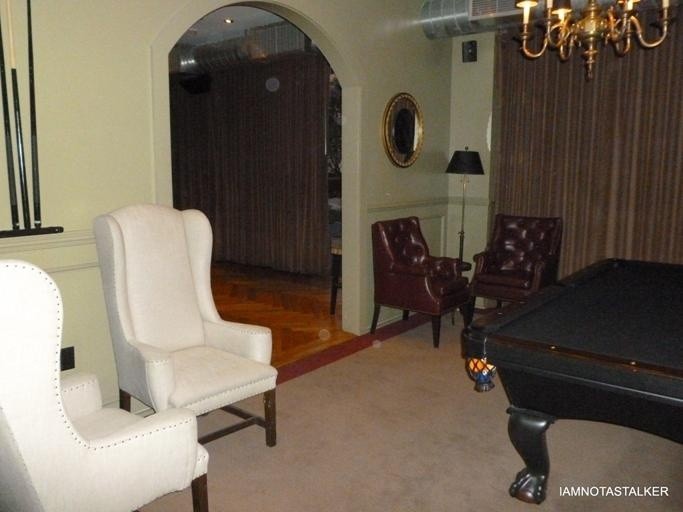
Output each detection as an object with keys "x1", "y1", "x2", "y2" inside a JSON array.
[{"x1": 0, "y1": 0, "x2": 41, "y2": 229}]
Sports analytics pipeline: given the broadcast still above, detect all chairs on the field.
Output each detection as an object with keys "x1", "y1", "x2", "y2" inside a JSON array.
[
  {"x1": 92, "y1": 203, "x2": 279, "y2": 447},
  {"x1": 469, "y1": 213, "x2": 563, "y2": 322},
  {"x1": 0, "y1": 260, "x2": 210, "y2": 512},
  {"x1": 370, "y1": 216, "x2": 476, "y2": 351}
]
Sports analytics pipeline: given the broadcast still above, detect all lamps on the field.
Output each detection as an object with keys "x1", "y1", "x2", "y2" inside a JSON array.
[
  {"x1": 445, "y1": 146, "x2": 485, "y2": 263},
  {"x1": 514, "y1": 0, "x2": 672, "y2": 81}
]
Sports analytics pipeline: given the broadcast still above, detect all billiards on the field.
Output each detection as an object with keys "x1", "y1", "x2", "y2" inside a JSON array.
[{"x1": 469, "y1": 356, "x2": 496, "y2": 382}]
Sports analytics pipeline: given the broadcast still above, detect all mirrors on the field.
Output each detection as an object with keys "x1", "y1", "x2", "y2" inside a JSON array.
[{"x1": 381, "y1": 92, "x2": 425, "y2": 168}]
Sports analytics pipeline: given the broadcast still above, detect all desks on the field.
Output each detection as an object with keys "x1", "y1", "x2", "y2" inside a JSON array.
[
  {"x1": 460, "y1": 257, "x2": 682, "y2": 504},
  {"x1": 329, "y1": 246, "x2": 342, "y2": 315}
]
